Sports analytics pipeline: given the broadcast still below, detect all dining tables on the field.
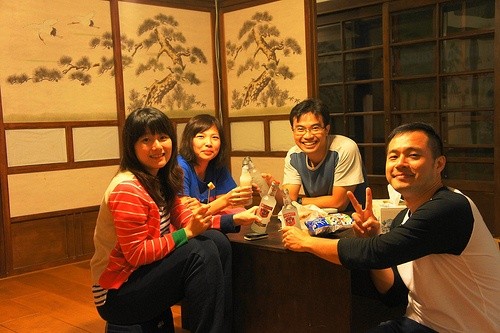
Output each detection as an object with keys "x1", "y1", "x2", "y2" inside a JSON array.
[{"x1": 181, "y1": 207, "x2": 410, "y2": 333}]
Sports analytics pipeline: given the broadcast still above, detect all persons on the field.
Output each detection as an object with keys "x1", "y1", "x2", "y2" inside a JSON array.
[
  {"x1": 282, "y1": 123, "x2": 500, "y2": 333},
  {"x1": 261, "y1": 99, "x2": 365, "y2": 213},
  {"x1": 89, "y1": 107, "x2": 262, "y2": 333},
  {"x1": 175, "y1": 114, "x2": 262, "y2": 217}
]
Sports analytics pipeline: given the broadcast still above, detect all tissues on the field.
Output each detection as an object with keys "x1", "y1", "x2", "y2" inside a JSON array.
[{"x1": 371, "y1": 181, "x2": 408, "y2": 234}]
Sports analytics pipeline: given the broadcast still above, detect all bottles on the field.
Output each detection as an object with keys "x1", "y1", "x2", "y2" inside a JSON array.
[
  {"x1": 247, "y1": 156, "x2": 269, "y2": 198},
  {"x1": 282, "y1": 188, "x2": 301, "y2": 229},
  {"x1": 250, "y1": 180, "x2": 280, "y2": 234},
  {"x1": 240, "y1": 158, "x2": 253, "y2": 206}
]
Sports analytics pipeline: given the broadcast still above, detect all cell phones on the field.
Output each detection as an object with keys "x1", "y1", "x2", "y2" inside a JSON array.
[{"x1": 244, "y1": 232, "x2": 268, "y2": 241}]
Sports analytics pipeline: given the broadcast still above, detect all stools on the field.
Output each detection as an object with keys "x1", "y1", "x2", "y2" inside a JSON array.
[{"x1": 105, "y1": 307, "x2": 174, "y2": 333}]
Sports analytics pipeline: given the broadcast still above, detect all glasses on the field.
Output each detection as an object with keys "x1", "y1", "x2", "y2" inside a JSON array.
[{"x1": 292, "y1": 125, "x2": 326, "y2": 135}]
